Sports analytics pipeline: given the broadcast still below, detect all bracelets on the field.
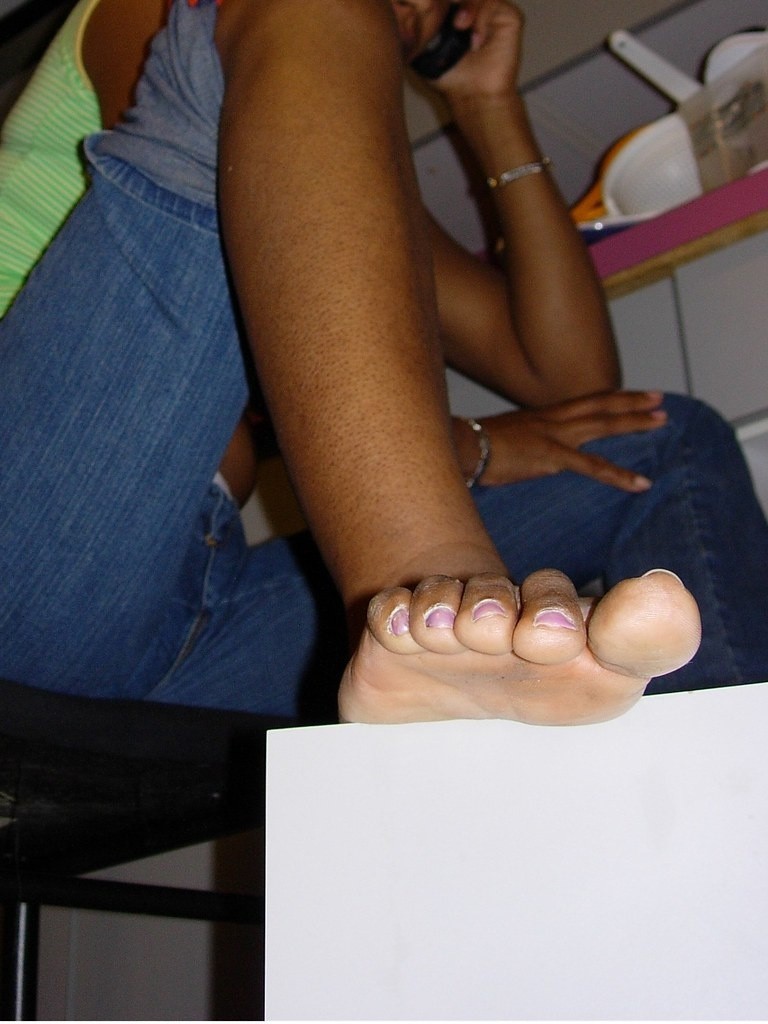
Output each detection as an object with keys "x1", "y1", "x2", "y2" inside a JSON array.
[
  {"x1": 453, "y1": 413, "x2": 490, "y2": 490},
  {"x1": 484, "y1": 156, "x2": 554, "y2": 203}
]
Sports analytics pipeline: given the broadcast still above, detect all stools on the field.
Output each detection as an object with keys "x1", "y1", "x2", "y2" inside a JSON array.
[{"x1": 0, "y1": 672, "x2": 334, "y2": 1021}]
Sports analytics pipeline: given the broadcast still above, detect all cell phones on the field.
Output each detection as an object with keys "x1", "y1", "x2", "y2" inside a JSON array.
[{"x1": 409, "y1": 0, "x2": 474, "y2": 79}]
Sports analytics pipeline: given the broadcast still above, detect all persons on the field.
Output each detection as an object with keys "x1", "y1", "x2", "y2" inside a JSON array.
[{"x1": 0, "y1": 0, "x2": 768, "y2": 726}]
